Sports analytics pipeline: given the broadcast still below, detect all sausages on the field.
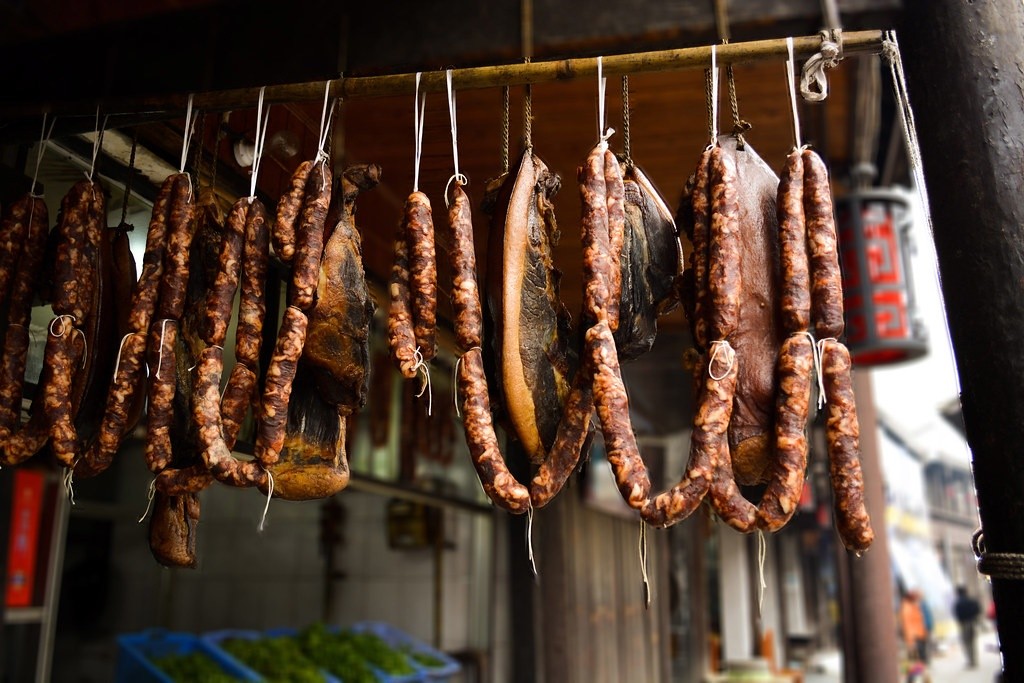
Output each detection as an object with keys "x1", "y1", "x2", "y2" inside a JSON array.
[{"x1": 0, "y1": 143, "x2": 875, "y2": 552}]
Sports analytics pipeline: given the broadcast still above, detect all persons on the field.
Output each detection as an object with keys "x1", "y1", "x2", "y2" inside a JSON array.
[
  {"x1": 951, "y1": 586, "x2": 979, "y2": 681},
  {"x1": 900, "y1": 592, "x2": 933, "y2": 683}
]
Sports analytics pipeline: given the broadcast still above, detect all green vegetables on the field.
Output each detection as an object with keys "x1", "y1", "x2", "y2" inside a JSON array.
[{"x1": 150, "y1": 624, "x2": 448, "y2": 683}]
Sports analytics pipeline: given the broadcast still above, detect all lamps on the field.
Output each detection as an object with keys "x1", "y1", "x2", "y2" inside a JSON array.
[{"x1": 836, "y1": 190, "x2": 933, "y2": 373}]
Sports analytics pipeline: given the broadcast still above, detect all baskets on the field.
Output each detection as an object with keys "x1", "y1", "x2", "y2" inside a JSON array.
[{"x1": 117, "y1": 621, "x2": 459, "y2": 682}]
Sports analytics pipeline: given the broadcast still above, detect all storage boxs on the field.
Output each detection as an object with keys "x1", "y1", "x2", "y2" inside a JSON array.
[{"x1": 115, "y1": 619, "x2": 461, "y2": 683}]
